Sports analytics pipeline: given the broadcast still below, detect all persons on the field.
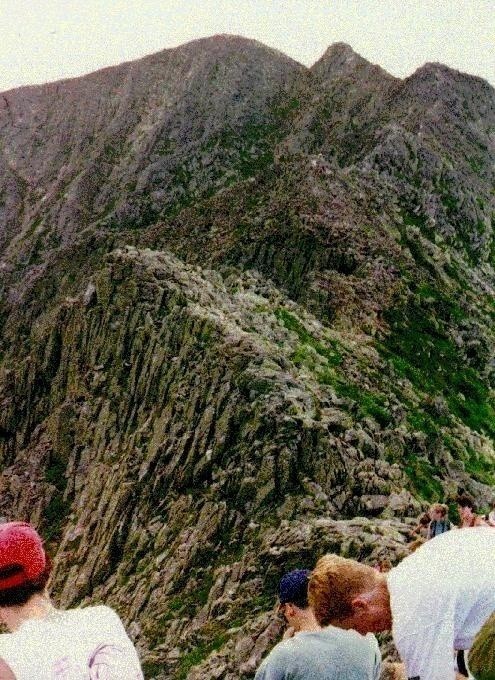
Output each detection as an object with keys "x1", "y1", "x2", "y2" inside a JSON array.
[
  {"x1": 253, "y1": 568, "x2": 383, "y2": 680},
  {"x1": 409, "y1": 496, "x2": 495, "y2": 551},
  {"x1": 307, "y1": 526, "x2": 495, "y2": 680},
  {"x1": 0, "y1": 521, "x2": 144, "y2": 680}
]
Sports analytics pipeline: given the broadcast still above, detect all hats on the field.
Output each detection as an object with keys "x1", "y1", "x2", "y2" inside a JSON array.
[
  {"x1": 0, "y1": 522, "x2": 45, "y2": 589},
  {"x1": 279, "y1": 570, "x2": 309, "y2": 606}
]
